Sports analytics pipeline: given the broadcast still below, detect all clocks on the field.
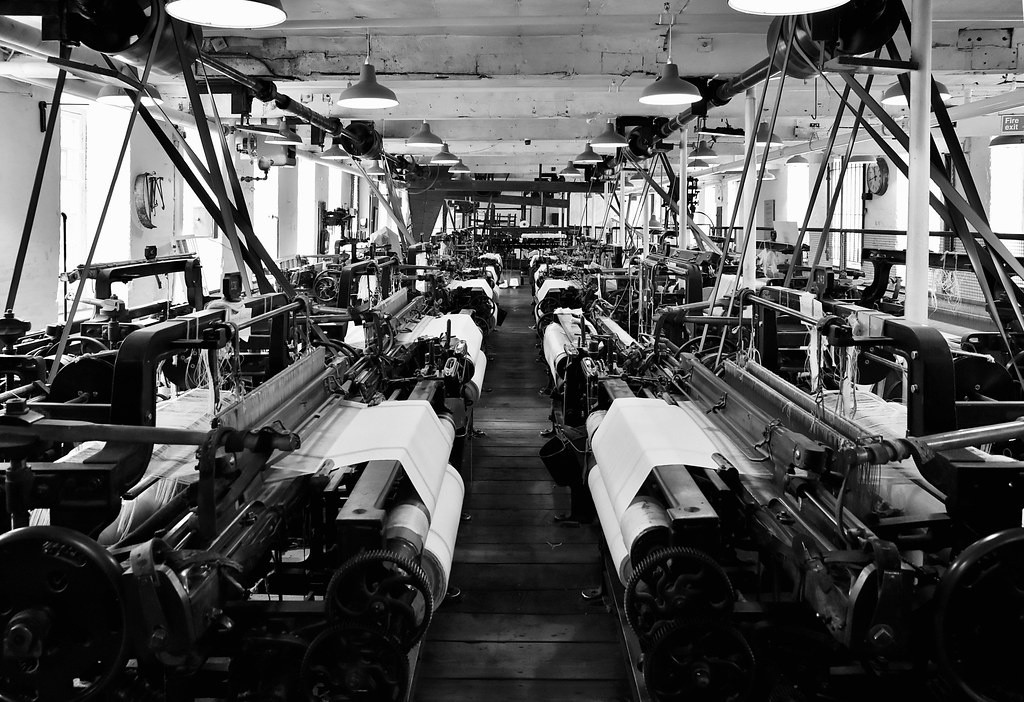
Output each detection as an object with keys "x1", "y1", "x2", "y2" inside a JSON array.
[{"x1": 866, "y1": 157, "x2": 889, "y2": 196}]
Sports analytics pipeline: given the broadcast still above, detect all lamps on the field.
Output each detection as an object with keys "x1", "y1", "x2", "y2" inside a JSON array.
[
  {"x1": 163, "y1": 0, "x2": 288, "y2": 29},
  {"x1": 429, "y1": 142, "x2": 459, "y2": 163},
  {"x1": 448, "y1": 158, "x2": 471, "y2": 173},
  {"x1": 558, "y1": 0, "x2": 954, "y2": 228},
  {"x1": 407, "y1": 118, "x2": 445, "y2": 148},
  {"x1": 93, "y1": 33, "x2": 400, "y2": 184}
]
